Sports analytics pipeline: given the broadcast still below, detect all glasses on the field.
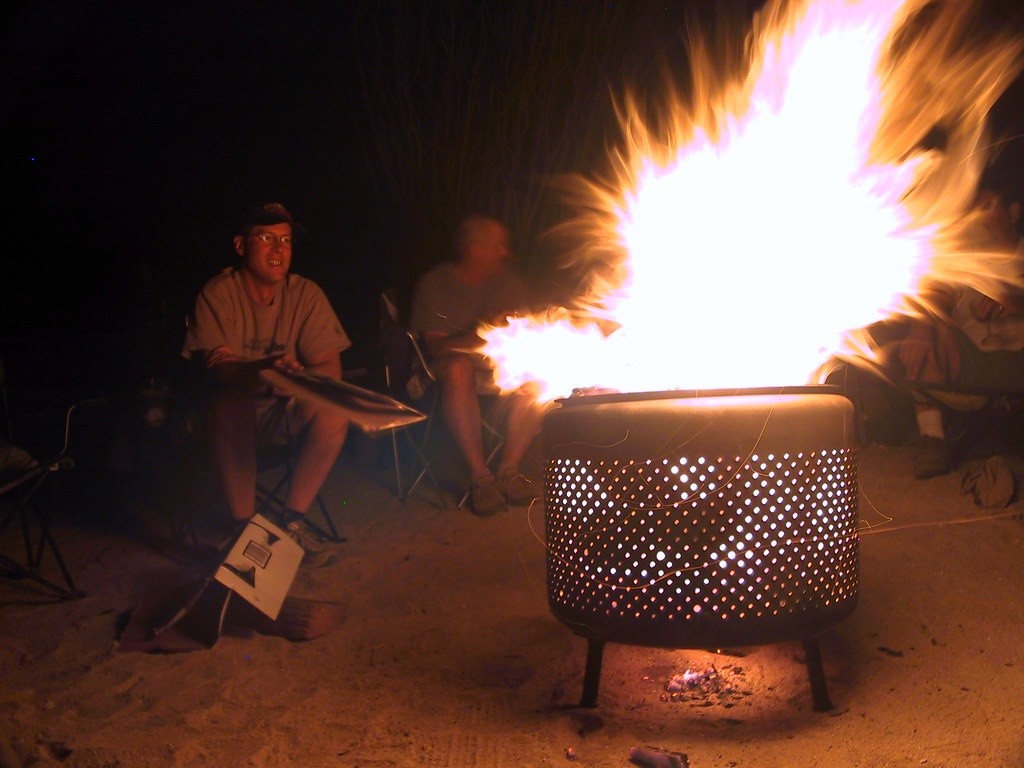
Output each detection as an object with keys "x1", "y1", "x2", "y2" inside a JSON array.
[{"x1": 246, "y1": 232, "x2": 292, "y2": 245}]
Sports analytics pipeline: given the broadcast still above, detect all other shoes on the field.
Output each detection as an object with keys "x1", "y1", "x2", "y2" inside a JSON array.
[
  {"x1": 913, "y1": 433, "x2": 960, "y2": 478},
  {"x1": 495, "y1": 466, "x2": 529, "y2": 506},
  {"x1": 279, "y1": 517, "x2": 325, "y2": 553},
  {"x1": 471, "y1": 479, "x2": 506, "y2": 516}
]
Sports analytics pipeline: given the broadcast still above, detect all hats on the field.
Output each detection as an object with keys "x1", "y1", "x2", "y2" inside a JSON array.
[{"x1": 240, "y1": 203, "x2": 305, "y2": 235}]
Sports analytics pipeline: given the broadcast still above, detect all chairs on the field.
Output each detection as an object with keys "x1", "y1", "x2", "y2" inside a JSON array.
[
  {"x1": 377, "y1": 284, "x2": 504, "y2": 510},
  {"x1": 0, "y1": 368, "x2": 111, "y2": 602},
  {"x1": 131, "y1": 314, "x2": 347, "y2": 564}
]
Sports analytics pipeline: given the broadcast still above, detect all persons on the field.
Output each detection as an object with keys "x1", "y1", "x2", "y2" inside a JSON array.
[
  {"x1": 901, "y1": 174, "x2": 1024, "y2": 479},
  {"x1": 409, "y1": 215, "x2": 549, "y2": 515},
  {"x1": 184, "y1": 200, "x2": 351, "y2": 568}
]
